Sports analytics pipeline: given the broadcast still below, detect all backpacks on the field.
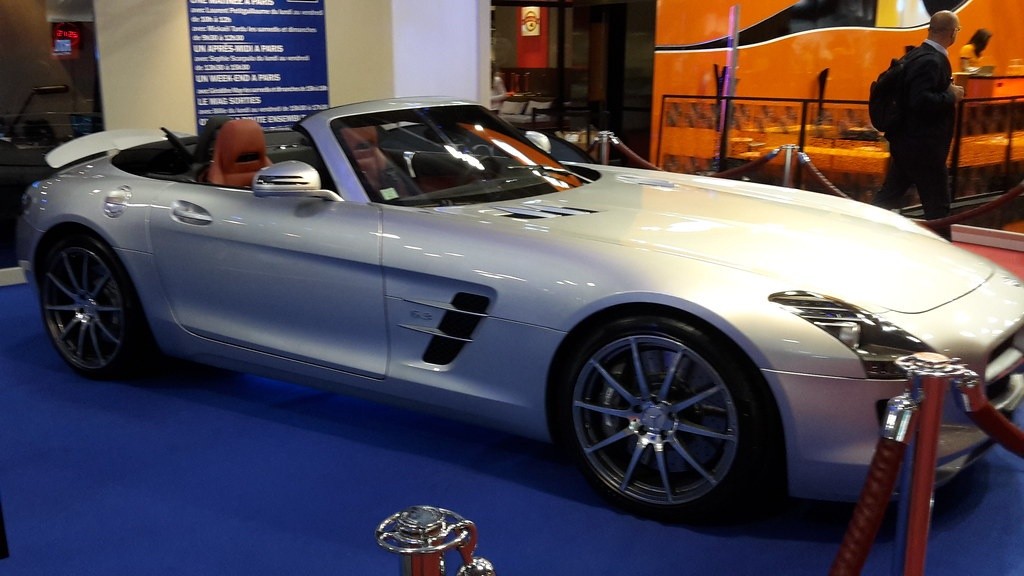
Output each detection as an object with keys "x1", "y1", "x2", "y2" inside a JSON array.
[{"x1": 867, "y1": 51, "x2": 938, "y2": 132}]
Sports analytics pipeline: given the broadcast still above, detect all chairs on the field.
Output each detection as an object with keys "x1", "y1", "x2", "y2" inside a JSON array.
[
  {"x1": 207, "y1": 119, "x2": 276, "y2": 191},
  {"x1": 343, "y1": 126, "x2": 425, "y2": 203}
]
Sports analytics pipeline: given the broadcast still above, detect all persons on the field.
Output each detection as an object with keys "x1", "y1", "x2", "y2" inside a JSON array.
[
  {"x1": 867, "y1": 10, "x2": 965, "y2": 241},
  {"x1": 490, "y1": 58, "x2": 507, "y2": 116},
  {"x1": 960, "y1": 28, "x2": 992, "y2": 71}
]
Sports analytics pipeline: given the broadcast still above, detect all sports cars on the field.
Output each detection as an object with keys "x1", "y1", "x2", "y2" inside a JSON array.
[{"x1": 15, "y1": 95, "x2": 1023, "y2": 520}]
